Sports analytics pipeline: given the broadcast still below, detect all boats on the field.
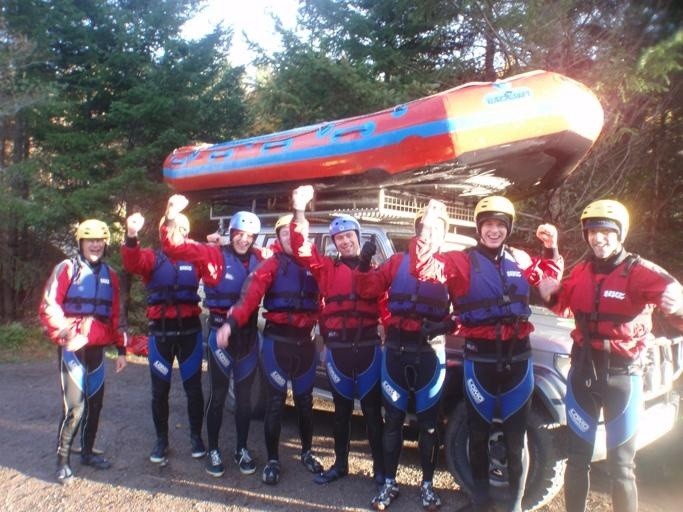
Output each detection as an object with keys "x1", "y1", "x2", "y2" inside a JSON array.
[{"x1": 160, "y1": 71, "x2": 606, "y2": 201}]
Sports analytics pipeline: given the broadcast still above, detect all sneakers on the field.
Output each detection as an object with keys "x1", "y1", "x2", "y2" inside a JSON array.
[
  {"x1": 419, "y1": 481, "x2": 443, "y2": 511},
  {"x1": 204, "y1": 448, "x2": 226, "y2": 477},
  {"x1": 189, "y1": 432, "x2": 207, "y2": 459},
  {"x1": 311, "y1": 463, "x2": 349, "y2": 486},
  {"x1": 261, "y1": 459, "x2": 282, "y2": 485},
  {"x1": 149, "y1": 437, "x2": 170, "y2": 464},
  {"x1": 79, "y1": 453, "x2": 112, "y2": 470},
  {"x1": 300, "y1": 449, "x2": 325, "y2": 475},
  {"x1": 370, "y1": 473, "x2": 399, "y2": 511},
  {"x1": 54, "y1": 461, "x2": 74, "y2": 486},
  {"x1": 230, "y1": 447, "x2": 258, "y2": 475}
]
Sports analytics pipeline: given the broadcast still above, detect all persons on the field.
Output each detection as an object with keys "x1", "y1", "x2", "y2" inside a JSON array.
[
  {"x1": 354, "y1": 206, "x2": 461, "y2": 511},
  {"x1": 290, "y1": 184, "x2": 390, "y2": 493},
  {"x1": 122, "y1": 212, "x2": 207, "y2": 463},
  {"x1": 39, "y1": 219, "x2": 128, "y2": 480},
  {"x1": 538, "y1": 198, "x2": 683, "y2": 512},
  {"x1": 163, "y1": 194, "x2": 274, "y2": 475},
  {"x1": 411, "y1": 198, "x2": 566, "y2": 512},
  {"x1": 215, "y1": 215, "x2": 325, "y2": 483}
]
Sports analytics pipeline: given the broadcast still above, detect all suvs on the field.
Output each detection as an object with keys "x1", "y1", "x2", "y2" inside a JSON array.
[{"x1": 192, "y1": 168, "x2": 683, "y2": 511}]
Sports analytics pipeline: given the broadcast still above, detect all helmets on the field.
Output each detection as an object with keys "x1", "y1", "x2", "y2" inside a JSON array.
[
  {"x1": 274, "y1": 214, "x2": 310, "y2": 254},
  {"x1": 413, "y1": 205, "x2": 449, "y2": 243},
  {"x1": 328, "y1": 215, "x2": 362, "y2": 253},
  {"x1": 228, "y1": 210, "x2": 261, "y2": 249},
  {"x1": 579, "y1": 198, "x2": 631, "y2": 244},
  {"x1": 75, "y1": 219, "x2": 111, "y2": 254},
  {"x1": 158, "y1": 212, "x2": 191, "y2": 241},
  {"x1": 473, "y1": 195, "x2": 515, "y2": 238}
]
